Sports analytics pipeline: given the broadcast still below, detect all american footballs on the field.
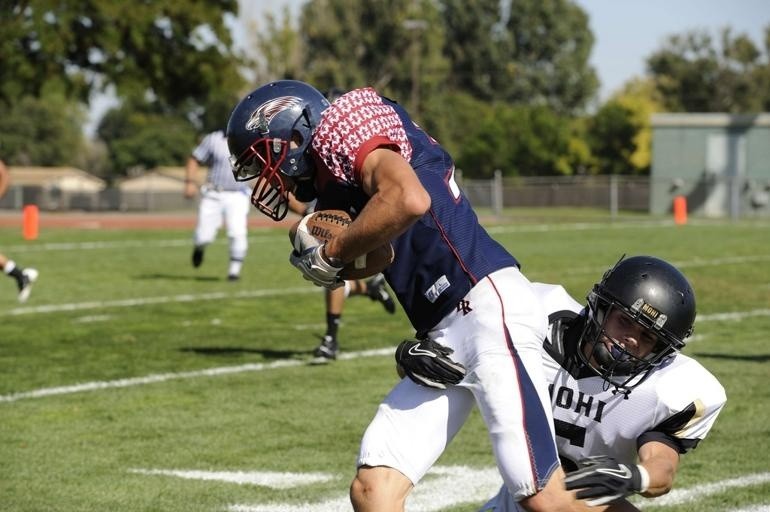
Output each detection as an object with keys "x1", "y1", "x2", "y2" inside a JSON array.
[{"x1": 290, "y1": 210, "x2": 395, "y2": 280}]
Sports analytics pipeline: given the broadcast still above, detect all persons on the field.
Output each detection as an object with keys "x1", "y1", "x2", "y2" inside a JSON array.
[
  {"x1": 224, "y1": 74, "x2": 609, "y2": 512},
  {"x1": 279, "y1": 181, "x2": 399, "y2": 363},
  {"x1": 393, "y1": 252, "x2": 728, "y2": 511},
  {"x1": 184, "y1": 127, "x2": 254, "y2": 280},
  {"x1": 0, "y1": 157, "x2": 41, "y2": 307}
]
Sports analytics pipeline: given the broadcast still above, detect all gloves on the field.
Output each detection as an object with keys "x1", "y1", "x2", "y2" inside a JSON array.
[
  {"x1": 563, "y1": 454, "x2": 651, "y2": 508},
  {"x1": 288, "y1": 243, "x2": 346, "y2": 291},
  {"x1": 393, "y1": 338, "x2": 467, "y2": 390}
]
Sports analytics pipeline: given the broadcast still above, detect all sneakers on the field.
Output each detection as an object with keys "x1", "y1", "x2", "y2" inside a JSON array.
[
  {"x1": 226, "y1": 257, "x2": 243, "y2": 280},
  {"x1": 367, "y1": 272, "x2": 396, "y2": 314},
  {"x1": 17, "y1": 267, "x2": 40, "y2": 304},
  {"x1": 308, "y1": 336, "x2": 340, "y2": 365},
  {"x1": 192, "y1": 246, "x2": 204, "y2": 268}
]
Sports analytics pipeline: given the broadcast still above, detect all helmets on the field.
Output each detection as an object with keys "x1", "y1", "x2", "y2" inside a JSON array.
[
  {"x1": 224, "y1": 78, "x2": 331, "y2": 203},
  {"x1": 589, "y1": 252, "x2": 696, "y2": 373}
]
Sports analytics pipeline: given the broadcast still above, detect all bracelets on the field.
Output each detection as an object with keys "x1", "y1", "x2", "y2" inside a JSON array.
[
  {"x1": 321, "y1": 239, "x2": 336, "y2": 269},
  {"x1": 633, "y1": 465, "x2": 651, "y2": 497}
]
